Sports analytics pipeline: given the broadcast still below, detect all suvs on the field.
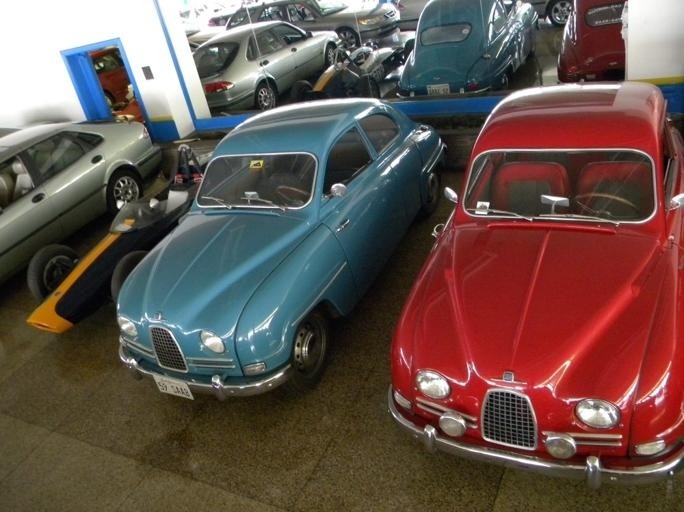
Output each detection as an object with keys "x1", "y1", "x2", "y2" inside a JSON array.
[{"x1": 226, "y1": 0, "x2": 400, "y2": 49}]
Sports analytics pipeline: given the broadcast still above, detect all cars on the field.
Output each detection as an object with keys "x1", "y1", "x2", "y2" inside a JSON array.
[
  {"x1": 524, "y1": 0, "x2": 575, "y2": 25},
  {"x1": 0, "y1": 120, "x2": 163, "y2": 282},
  {"x1": 180, "y1": 26, "x2": 227, "y2": 52},
  {"x1": 194, "y1": 21, "x2": 343, "y2": 110},
  {"x1": 385, "y1": 81, "x2": 684, "y2": 485},
  {"x1": 208, "y1": 8, "x2": 240, "y2": 26},
  {"x1": 90, "y1": 48, "x2": 132, "y2": 108},
  {"x1": 557, "y1": 0, "x2": 626, "y2": 82},
  {"x1": 115, "y1": 96, "x2": 448, "y2": 404},
  {"x1": 398, "y1": 0, "x2": 539, "y2": 97}
]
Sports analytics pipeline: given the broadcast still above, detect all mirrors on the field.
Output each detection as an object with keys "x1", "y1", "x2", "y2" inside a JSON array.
[{"x1": 175, "y1": 0, "x2": 629, "y2": 118}]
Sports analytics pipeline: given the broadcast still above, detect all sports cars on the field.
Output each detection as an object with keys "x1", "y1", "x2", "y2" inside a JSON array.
[
  {"x1": 291, "y1": 40, "x2": 416, "y2": 97},
  {"x1": 27, "y1": 142, "x2": 271, "y2": 338}
]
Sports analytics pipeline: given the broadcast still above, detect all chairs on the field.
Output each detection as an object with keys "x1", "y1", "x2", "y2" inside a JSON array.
[
  {"x1": 570, "y1": 159, "x2": 654, "y2": 222},
  {"x1": 256, "y1": 115, "x2": 396, "y2": 206},
  {"x1": 486, "y1": 160, "x2": 570, "y2": 219},
  {"x1": 0, "y1": 159, "x2": 32, "y2": 209}
]
